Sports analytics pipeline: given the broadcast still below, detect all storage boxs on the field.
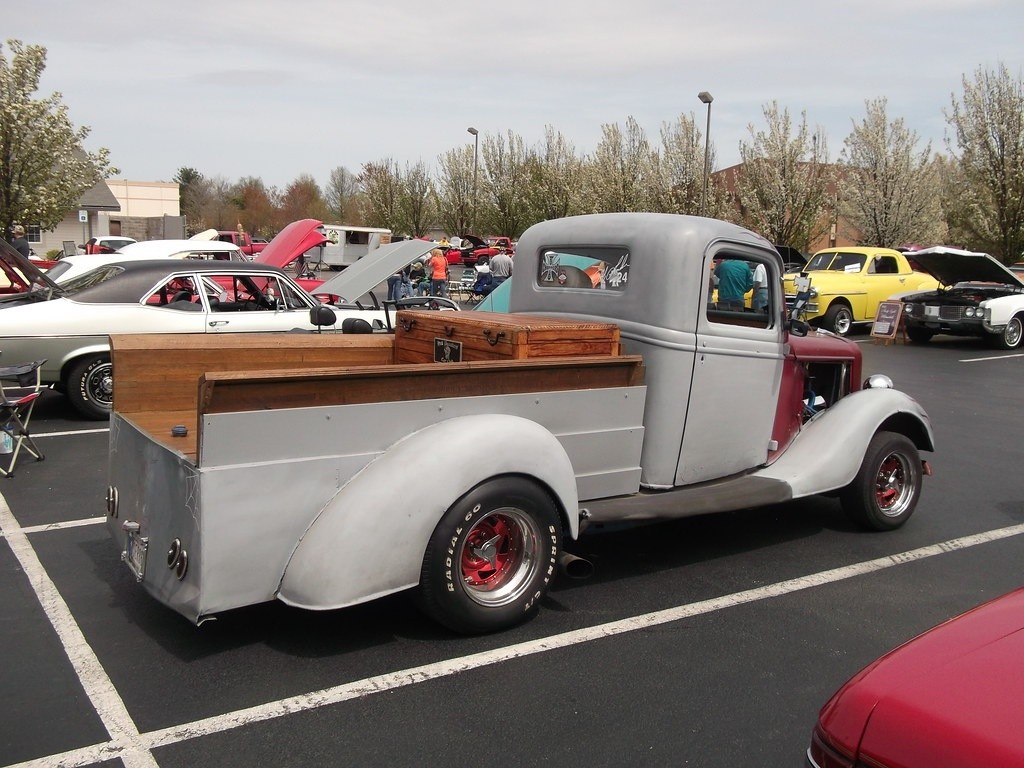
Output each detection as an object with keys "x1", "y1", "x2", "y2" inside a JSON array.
[{"x1": 393, "y1": 308, "x2": 621, "y2": 366}]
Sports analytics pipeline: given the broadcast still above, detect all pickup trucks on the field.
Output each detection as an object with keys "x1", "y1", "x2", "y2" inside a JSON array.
[{"x1": 106, "y1": 211, "x2": 933, "y2": 638}]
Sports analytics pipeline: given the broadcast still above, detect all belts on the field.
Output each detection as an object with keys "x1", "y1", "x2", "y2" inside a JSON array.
[{"x1": 759, "y1": 287, "x2": 768, "y2": 289}]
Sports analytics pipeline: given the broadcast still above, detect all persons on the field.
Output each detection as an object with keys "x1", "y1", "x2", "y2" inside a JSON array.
[
  {"x1": 440, "y1": 237, "x2": 449, "y2": 246},
  {"x1": 409, "y1": 235, "x2": 419, "y2": 240},
  {"x1": 708, "y1": 260, "x2": 753, "y2": 312},
  {"x1": 388, "y1": 249, "x2": 449, "y2": 308},
  {"x1": 490, "y1": 247, "x2": 515, "y2": 286},
  {"x1": 752, "y1": 264, "x2": 768, "y2": 313},
  {"x1": 8, "y1": 226, "x2": 29, "y2": 265}
]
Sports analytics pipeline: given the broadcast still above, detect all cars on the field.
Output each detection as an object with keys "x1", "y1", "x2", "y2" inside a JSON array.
[
  {"x1": 807, "y1": 586, "x2": 1024, "y2": 768},
  {"x1": 891, "y1": 245, "x2": 1024, "y2": 350},
  {"x1": 0, "y1": 260, "x2": 462, "y2": 421},
  {"x1": 0, "y1": 206, "x2": 520, "y2": 309},
  {"x1": 710, "y1": 247, "x2": 955, "y2": 338}
]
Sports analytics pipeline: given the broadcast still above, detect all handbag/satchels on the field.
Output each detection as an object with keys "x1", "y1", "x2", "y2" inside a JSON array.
[{"x1": 444, "y1": 257, "x2": 450, "y2": 276}]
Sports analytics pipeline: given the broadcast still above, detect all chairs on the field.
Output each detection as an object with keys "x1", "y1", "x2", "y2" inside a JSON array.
[
  {"x1": 424, "y1": 280, "x2": 485, "y2": 306},
  {"x1": 170, "y1": 291, "x2": 192, "y2": 305},
  {"x1": 195, "y1": 295, "x2": 219, "y2": 313},
  {"x1": 0, "y1": 359, "x2": 55, "y2": 480},
  {"x1": 540, "y1": 265, "x2": 593, "y2": 288}
]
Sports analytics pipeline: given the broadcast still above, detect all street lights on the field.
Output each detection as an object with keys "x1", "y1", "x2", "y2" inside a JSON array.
[
  {"x1": 698, "y1": 90, "x2": 714, "y2": 217},
  {"x1": 467, "y1": 127, "x2": 478, "y2": 234}
]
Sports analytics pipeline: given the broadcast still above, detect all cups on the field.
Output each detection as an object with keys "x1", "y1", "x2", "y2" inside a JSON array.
[{"x1": 0, "y1": 424, "x2": 14, "y2": 455}]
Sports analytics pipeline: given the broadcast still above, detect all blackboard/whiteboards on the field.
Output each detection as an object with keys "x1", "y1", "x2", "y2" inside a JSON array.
[{"x1": 870, "y1": 299, "x2": 904, "y2": 339}]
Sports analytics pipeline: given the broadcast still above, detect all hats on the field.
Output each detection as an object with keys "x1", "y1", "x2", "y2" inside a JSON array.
[
  {"x1": 412, "y1": 262, "x2": 422, "y2": 267},
  {"x1": 499, "y1": 247, "x2": 506, "y2": 251},
  {"x1": 12, "y1": 226, "x2": 24, "y2": 233}
]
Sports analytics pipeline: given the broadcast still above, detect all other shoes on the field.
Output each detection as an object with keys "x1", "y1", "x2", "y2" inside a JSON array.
[
  {"x1": 424, "y1": 302, "x2": 429, "y2": 307},
  {"x1": 414, "y1": 305, "x2": 420, "y2": 308}
]
